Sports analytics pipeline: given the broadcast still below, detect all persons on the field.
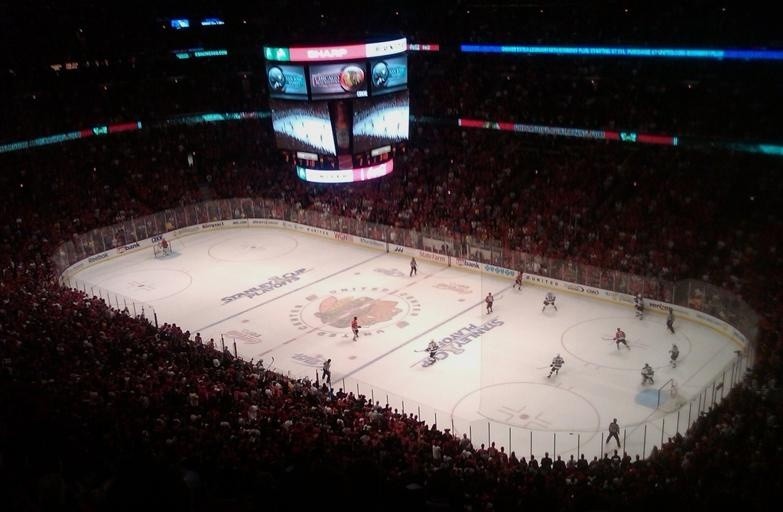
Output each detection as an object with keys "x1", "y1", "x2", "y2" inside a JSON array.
[
  {"x1": 542, "y1": 292, "x2": 558, "y2": 311},
  {"x1": 548, "y1": 355, "x2": 565, "y2": 378},
  {"x1": 304, "y1": 380, "x2": 606, "y2": 512},
  {"x1": 605, "y1": 289, "x2": 781, "y2": 512},
  {"x1": 322, "y1": 359, "x2": 332, "y2": 383},
  {"x1": 426, "y1": 340, "x2": 438, "y2": 362},
  {"x1": 1, "y1": 1, "x2": 781, "y2": 292},
  {"x1": 2, "y1": 261, "x2": 309, "y2": 511},
  {"x1": 352, "y1": 317, "x2": 362, "y2": 342},
  {"x1": 486, "y1": 293, "x2": 494, "y2": 314}
]
